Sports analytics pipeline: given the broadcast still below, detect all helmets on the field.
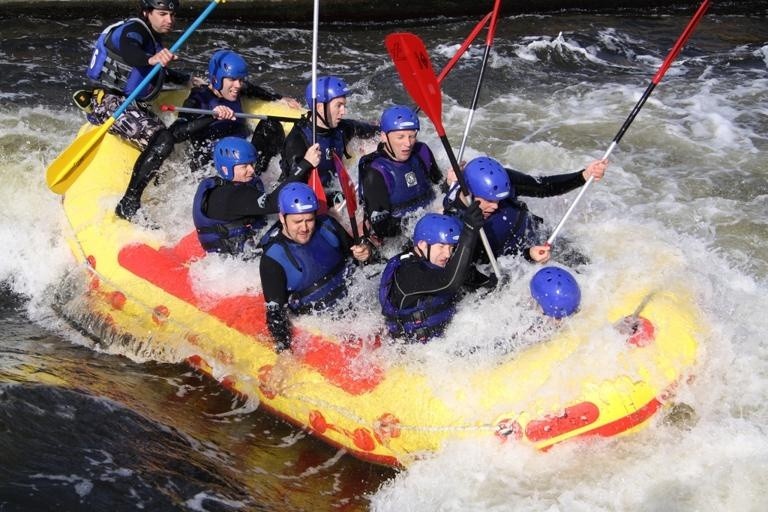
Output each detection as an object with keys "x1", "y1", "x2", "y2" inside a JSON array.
[
  {"x1": 138, "y1": 1, "x2": 178, "y2": 11},
  {"x1": 530, "y1": 267, "x2": 581, "y2": 317},
  {"x1": 414, "y1": 214, "x2": 462, "y2": 245},
  {"x1": 213, "y1": 137, "x2": 258, "y2": 181},
  {"x1": 462, "y1": 157, "x2": 511, "y2": 201},
  {"x1": 380, "y1": 107, "x2": 420, "y2": 134},
  {"x1": 208, "y1": 51, "x2": 248, "y2": 91},
  {"x1": 305, "y1": 77, "x2": 351, "y2": 109},
  {"x1": 278, "y1": 183, "x2": 318, "y2": 214}
]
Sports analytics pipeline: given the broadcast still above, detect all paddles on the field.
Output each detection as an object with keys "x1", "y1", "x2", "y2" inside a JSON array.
[
  {"x1": 386, "y1": 32, "x2": 505, "y2": 281},
  {"x1": 540, "y1": 0, "x2": 713, "y2": 255},
  {"x1": 332, "y1": 151, "x2": 364, "y2": 268},
  {"x1": 46, "y1": 0, "x2": 225, "y2": 195},
  {"x1": 307, "y1": 0, "x2": 328, "y2": 216},
  {"x1": 415, "y1": 12, "x2": 492, "y2": 114},
  {"x1": 457, "y1": 1, "x2": 503, "y2": 170}
]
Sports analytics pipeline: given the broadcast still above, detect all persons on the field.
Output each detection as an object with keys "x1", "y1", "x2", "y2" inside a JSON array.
[
  {"x1": 168, "y1": 49, "x2": 285, "y2": 178},
  {"x1": 529, "y1": 264, "x2": 582, "y2": 321},
  {"x1": 259, "y1": 181, "x2": 382, "y2": 354},
  {"x1": 441, "y1": 156, "x2": 612, "y2": 275},
  {"x1": 72, "y1": 0, "x2": 180, "y2": 233},
  {"x1": 283, "y1": 73, "x2": 383, "y2": 252},
  {"x1": 191, "y1": 137, "x2": 322, "y2": 262},
  {"x1": 358, "y1": 104, "x2": 468, "y2": 239},
  {"x1": 378, "y1": 199, "x2": 509, "y2": 347}
]
